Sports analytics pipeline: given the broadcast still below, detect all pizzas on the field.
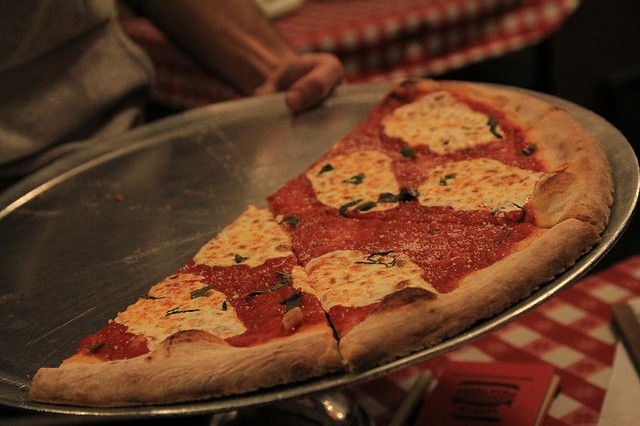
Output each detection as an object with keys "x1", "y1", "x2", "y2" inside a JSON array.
[{"x1": 29, "y1": 78, "x2": 614, "y2": 406}]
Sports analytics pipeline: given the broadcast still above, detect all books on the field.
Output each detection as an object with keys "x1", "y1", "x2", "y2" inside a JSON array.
[{"x1": 417, "y1": 361, "x2": 561, "y2": 425}]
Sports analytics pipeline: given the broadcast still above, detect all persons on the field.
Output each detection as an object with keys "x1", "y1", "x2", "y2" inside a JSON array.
[{"x1": 0, "y1": 0, "x2": 347, "y2": 184}]
